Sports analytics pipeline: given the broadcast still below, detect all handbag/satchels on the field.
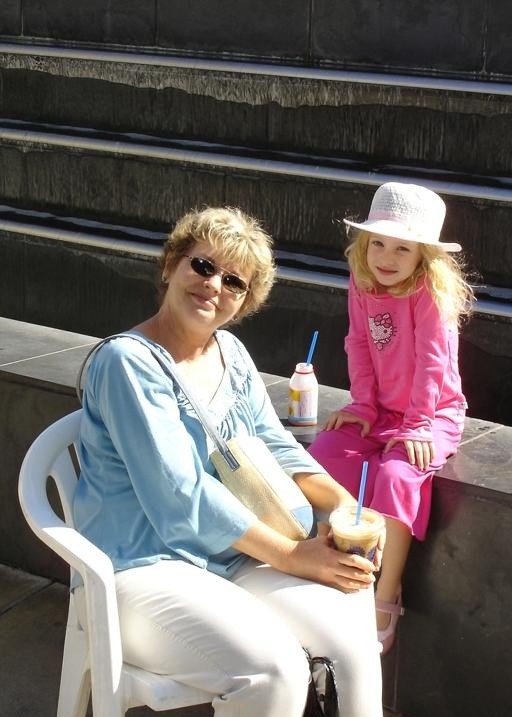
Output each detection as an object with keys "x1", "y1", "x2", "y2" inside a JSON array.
[{"x1": 211, "y1": 434, "x2": 323, "y2": 541}]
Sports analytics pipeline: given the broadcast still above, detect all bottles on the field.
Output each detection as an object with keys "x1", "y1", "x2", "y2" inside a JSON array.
[{"x1": 287, "y1": 360, "x2": 322, "y2": 428}]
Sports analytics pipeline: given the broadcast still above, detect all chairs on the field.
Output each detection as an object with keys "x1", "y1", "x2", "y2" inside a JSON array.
[{"x1": 19, "y1": 407, "x2": 219, "y2": 717}]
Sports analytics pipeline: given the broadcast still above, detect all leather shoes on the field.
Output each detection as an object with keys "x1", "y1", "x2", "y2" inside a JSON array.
[{"x1": 377, "y1": 593, "x2": 406, "y2": 656}]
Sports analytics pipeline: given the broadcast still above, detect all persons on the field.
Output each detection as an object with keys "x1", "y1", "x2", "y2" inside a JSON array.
[
  {"x1": 300, "y1": 180, "x2": 484, "y2": 658},
  {"x1": 70, "y1": 201, "x2": 391, "y2": 716}
]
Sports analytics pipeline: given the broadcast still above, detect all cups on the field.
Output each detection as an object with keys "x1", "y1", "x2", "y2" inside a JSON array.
[{"x1": 326, "y1": 503, "x2": 387, "y2": 576}]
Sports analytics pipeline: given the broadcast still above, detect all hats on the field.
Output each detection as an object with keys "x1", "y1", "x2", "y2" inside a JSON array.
[{"x1": 343, "y1": 182, "x2": 463, "y2": 254}]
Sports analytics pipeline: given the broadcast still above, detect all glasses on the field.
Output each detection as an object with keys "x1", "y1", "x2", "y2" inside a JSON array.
[{"x1": 179, "y1": 253, "x2": 252, "y2": 295}]
